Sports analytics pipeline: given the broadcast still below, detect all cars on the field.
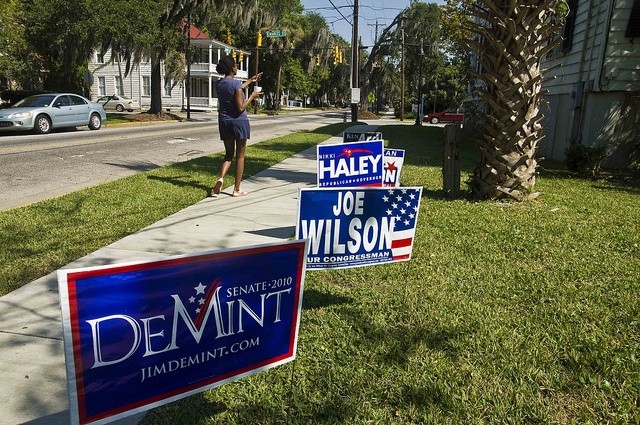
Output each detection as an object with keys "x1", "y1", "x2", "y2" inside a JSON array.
[
  {"x1": 96, "y1": 95, "x2": 138, "y2": 111},
  {"x1": 423, "y1": 108, "x2": 464, "y2": 124},
  {"x1": 0, "y1": 93, "x2": 107, "y2": 133}
]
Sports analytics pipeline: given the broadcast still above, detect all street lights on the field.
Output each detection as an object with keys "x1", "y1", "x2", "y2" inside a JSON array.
[
  {"x1": 398, "y1": 30, "x2": 408, "y2": 121},
  {"x1": 415, "y1": 39, "x2": 425, "y2": 126}
]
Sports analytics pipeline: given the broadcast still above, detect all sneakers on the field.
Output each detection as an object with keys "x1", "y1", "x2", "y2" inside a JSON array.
[
  {"x1": 233, "y1": 190, "x2": 248, "y2": 196},
  {"x1": 214, "y1": 177, "x2": 223, "y2": 194}
]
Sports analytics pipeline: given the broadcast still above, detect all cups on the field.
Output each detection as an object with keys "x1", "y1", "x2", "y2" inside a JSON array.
[{"x1": 254, "y1": 86, "x2": 262, "y2": 101}]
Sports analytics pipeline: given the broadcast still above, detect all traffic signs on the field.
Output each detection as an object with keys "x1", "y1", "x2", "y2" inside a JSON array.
[{"x1": 267, "y1": 29, "x2": 286, "y2": 38}]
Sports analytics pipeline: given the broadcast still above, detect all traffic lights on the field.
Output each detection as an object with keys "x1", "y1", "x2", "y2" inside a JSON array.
[
  {"x1": 332, "y1": 51, "x2": 336, "y2": 65},
  {"x1": 238, "y1": 52, "x2": 243, "y2": 62},
  {"x1": 232, "y1": 49, "x2": 236, "y2": 58},
  {"x1": 316, "y1": 54, "x2": 321, "y2": 66}
]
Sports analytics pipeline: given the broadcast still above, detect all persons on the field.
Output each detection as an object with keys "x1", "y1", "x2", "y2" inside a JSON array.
[{"x1": 214, "y1": 56, "x2": 264, "y2": 196}]
